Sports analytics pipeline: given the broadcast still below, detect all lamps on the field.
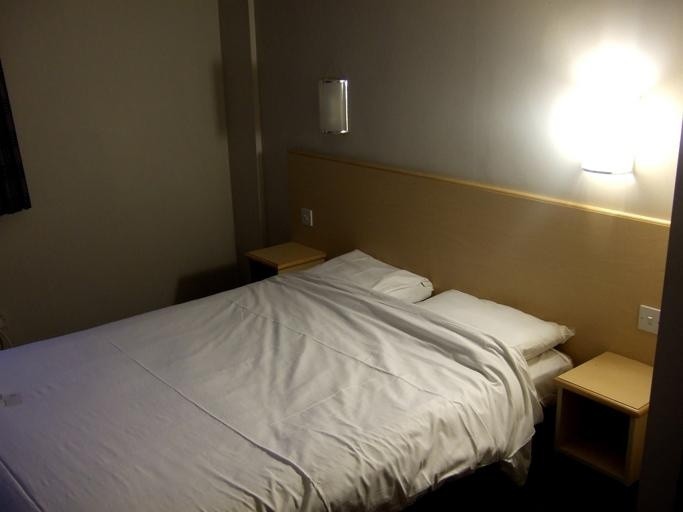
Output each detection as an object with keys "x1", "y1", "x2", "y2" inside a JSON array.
[{"x1": 318, "y1": 74, "x2": 350, "y2": 135}]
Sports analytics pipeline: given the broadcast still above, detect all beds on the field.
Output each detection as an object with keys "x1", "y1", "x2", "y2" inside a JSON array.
[{"x1": 1, "y1": 247, "x2": 573, "y2": 512}]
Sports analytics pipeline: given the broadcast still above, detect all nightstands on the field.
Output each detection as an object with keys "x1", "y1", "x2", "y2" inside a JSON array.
[
  {"x1": 245, "y1": 243, "x2": 326, "y2": 285},
  {"x1": 548, "y1": 348, "x2": 655, "y2": 490}
]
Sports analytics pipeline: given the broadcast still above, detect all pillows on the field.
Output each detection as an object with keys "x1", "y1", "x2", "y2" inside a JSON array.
[{"x1": 303, "y1": 251, "x2": 571, "y2": 365}]
